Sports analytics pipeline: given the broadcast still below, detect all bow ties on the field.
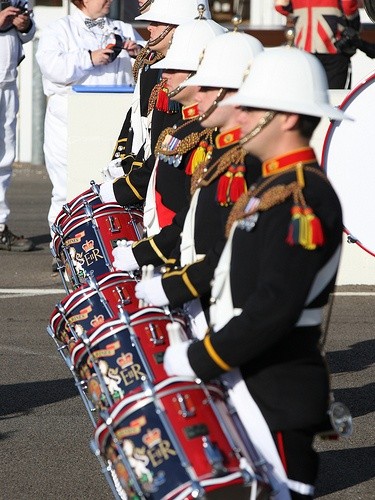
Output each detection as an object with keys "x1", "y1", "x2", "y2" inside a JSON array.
[{"x1": 85, "y1": 18, "x2": 105, "y2": 29}]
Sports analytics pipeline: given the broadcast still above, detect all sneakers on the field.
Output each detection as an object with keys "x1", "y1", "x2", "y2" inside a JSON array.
[{"x1": 0, "y1": 224, "x2": 35, "y2": 251}]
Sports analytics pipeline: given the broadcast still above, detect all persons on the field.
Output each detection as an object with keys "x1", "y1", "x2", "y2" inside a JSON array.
[
  {"x1": 273, "y1": 1, "x2": 361, "y2": 89},
  {"x1": 163, "y1": 47, "x2": 354, "y2": 500},
  {"x1": 0, "y1": 0, "x2": 36, "y2": 252},
  {"x1": 99, "y1": 0, "x2": 264, "y2": 342},
  {"x1": 36, "y1": 0, "x2": 148, "y2": 272}
]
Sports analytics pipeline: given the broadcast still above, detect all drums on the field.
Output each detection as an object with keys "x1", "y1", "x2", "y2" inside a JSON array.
[
  {"x1": 93, "y1": 375, "x2": 278, "y2": 500},
  {"x1": 52, "y1": 180, "x2": 102, "y2": 234},
  {"x1": 47, "y1": 271, "x2": 153, "y2": 356},
  {"x1": 320, "y1": 72, "x2": 375, "y2": 257},
  {"x1": 69, "y1": 307, "x2": 187, "y2": 416},
  {"x1": 50, "y1": 202, "x2": 146, "y2": 291}
]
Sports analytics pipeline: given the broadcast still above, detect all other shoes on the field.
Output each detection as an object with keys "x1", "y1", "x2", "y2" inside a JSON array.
[{"x1": 51, "y1": 258, "x2": 65, "y2": 272}]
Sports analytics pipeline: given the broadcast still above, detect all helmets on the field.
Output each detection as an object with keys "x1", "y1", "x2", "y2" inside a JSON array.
[
  {"x1": 218, "y1": 48, "x2": 347, "y2": 120},
  {"x1": 135, "y1": 0, "x2": 211, "y2": 25},
  {"x1": 180, "y1": 32, "x2": 264, "y2": 88},
  {"x1": 150, "y1": 20, "x2": 229, "y2": 71}
]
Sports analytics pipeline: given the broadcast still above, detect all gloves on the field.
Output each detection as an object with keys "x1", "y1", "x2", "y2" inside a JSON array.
[
  {"x1": 108, "y1": 158, "x2": 125, "y2": 178},
  {"x1": 135, "y1": 274, "x2": 169, "y2": 307},
  {"x1": 163, "y1": 340, "x2": 198, "y2": 379},
  {"x1": 99, "y1": 182, "x2": 117, "y2": 204},
  {"x1": 112, "y1": 241, "x2": 140, "y2": 271}
]
